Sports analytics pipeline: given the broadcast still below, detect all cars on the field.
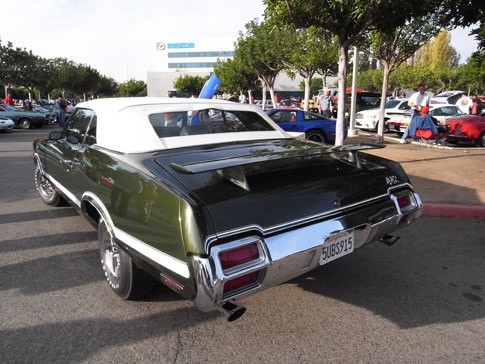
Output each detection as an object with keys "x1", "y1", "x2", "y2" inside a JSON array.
[
  {"x1": 251, "y1": 99, "x2": 315, "y2": 110},
  {"x1": 232, "y1": 108, "x2": 347, "y2": 146},
  {"x1": 30, "y1": 97, "x2": 425, "y2": 319},
  {"x1": 199, "y1": 111, "x2": 239, "y2": 126},
  {"x1": 0, "y1": 103, "x2": 57, "y2": 129},
  {"x1": 0, "y1": 116, "x2": 15, "y2": 130},
  {"x1": 316, "y1": 86, "x2": 485, "y2": 146}
]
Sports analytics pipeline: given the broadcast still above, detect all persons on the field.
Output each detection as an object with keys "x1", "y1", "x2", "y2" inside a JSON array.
[
  {"x1": 455, "y1": 92, "x2": 484, "y2": 116},
  {"x1": 4, "y1": 94, "x2": 15, "y2": 107},
  {"x1": 56, "y1": 94, "x2": 67, "y2": 127},
  {"x1": 274, "y1": 93, "x2": 281, "y2": 109},
  {"x1": 23, "y1": 99, "x2": 32, "y2": 111},
  {"x1": 318, "y1": 90, "x2": 338, "y2": 120},
  {"x1": 400, "y1": 84, "x2": 448, "y2": 146}
]
